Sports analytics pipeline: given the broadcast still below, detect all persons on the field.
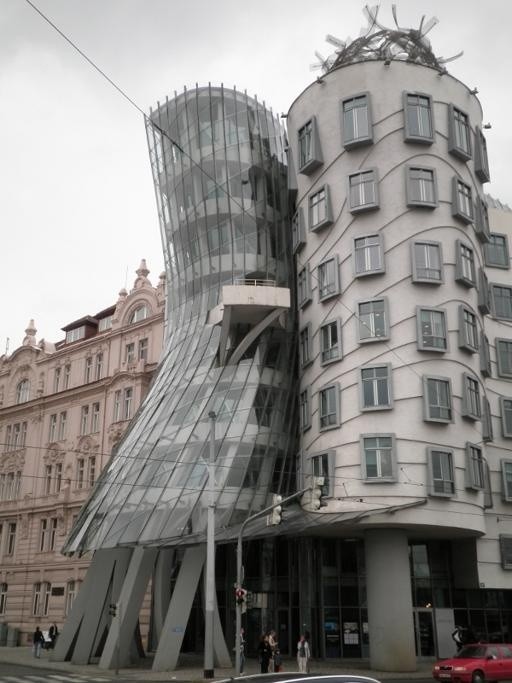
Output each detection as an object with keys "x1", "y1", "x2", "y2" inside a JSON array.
[
  {"x1": 451, "y1": 623, "x2": 480, "y2": 656},
  {"x1": 232, "y1": 626, "x2": 312, "y2": 676},
  {"x1": 33, "y1": 626, "x2": 45, "y2": 659},
  {"x1": 46, "y1": 621, "x2": 60, "y2": 652}
]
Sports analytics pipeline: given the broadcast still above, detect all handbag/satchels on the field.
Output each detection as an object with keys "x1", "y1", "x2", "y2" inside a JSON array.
[
  {"x1": 273, "y1": 649, "x2": 284, "y2": 667},
  {"x1": 258, "y1": 649, "x2": 263, "y2": 664},
  {"x1": 299, "y1": 639, "x2": 307, "y2": 658}
]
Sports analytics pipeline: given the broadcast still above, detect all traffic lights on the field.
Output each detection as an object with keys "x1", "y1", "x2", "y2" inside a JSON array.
[
  {"x1": 242, "y1": 589, "x2": 255, "y2": 613},
  {"x1": 272, "y1": 492, "x2": 287, "y2": 525},
  {"x1": 311, "y1": 475, "x2": 329, "y2": 511},
  {"x1": 235, "y1": 587, "x2": 245, "y2": 604},
  {"x1": 107, "y1": 602, "x2": 117, "y2": 617}
]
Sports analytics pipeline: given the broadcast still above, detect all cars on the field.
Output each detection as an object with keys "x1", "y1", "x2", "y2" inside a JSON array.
[
  {"x1": 431, "y1": 642, "x2": 512, "y2": 682},
  {"x1": 210, "y1": 670, "x2": 382, "y2": 682}
]
